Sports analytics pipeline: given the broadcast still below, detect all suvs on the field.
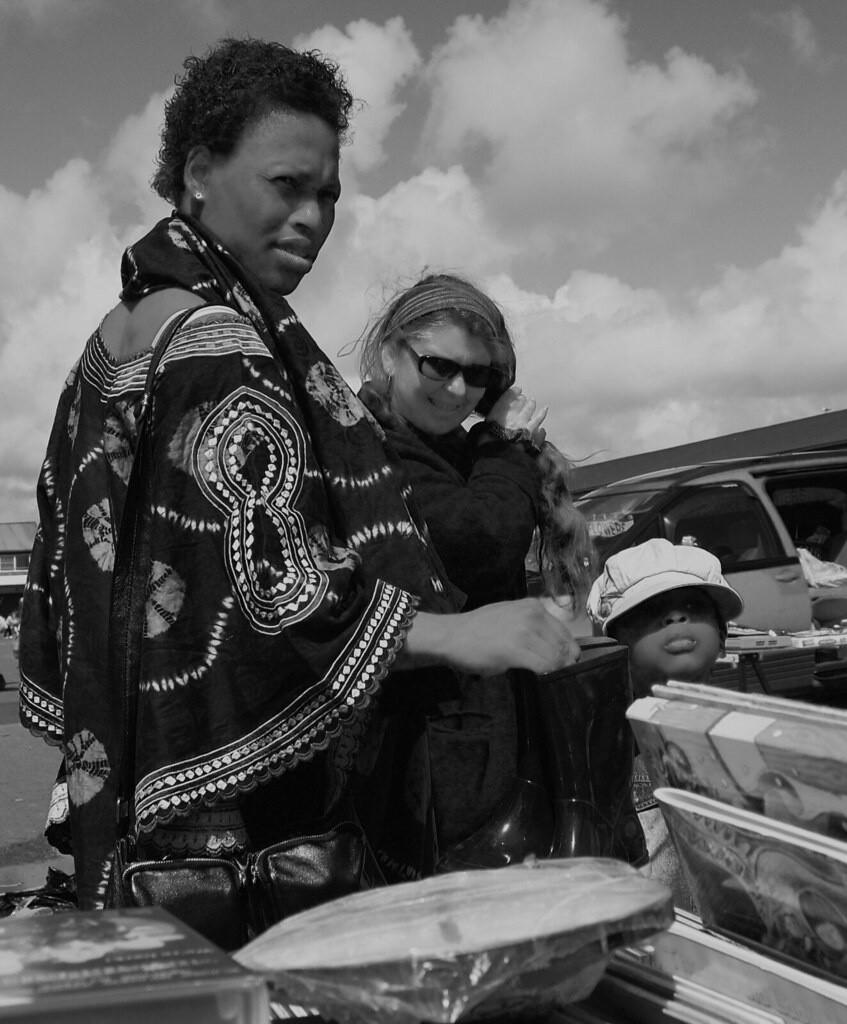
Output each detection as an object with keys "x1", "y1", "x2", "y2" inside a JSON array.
[{"x1": 566, "y1": 445, "x2": 846, "y2": 646}]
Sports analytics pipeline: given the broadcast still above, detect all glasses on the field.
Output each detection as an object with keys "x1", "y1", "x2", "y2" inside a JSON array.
[{"x1": 399, "y1": 337, "x2": 504, "y2": 389}]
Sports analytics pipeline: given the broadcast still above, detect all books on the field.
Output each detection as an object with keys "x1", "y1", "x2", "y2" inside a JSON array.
[{"x1": 625, "y1": 679, "x2": 846, "y2": 988}]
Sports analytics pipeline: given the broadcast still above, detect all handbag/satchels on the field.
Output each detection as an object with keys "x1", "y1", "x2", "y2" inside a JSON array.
[
  {"x1": 113, "y1": 817, "x2": 390, "y2": 957},
  {"x1": 420, "y1": 769, "x2": 605, "y2": 876}
]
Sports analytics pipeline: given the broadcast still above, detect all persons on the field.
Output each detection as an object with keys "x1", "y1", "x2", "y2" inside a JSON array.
[
  {"x1": 586, "y1": 537, "x2": 743, "y2": 681},
  {"x1": 18, "y1": 36, "x2": 582, "y2": 949},
  {"x1": 338, "y1": 273, "x2": 595, "y2": 839},
  {"x1": 3, "y1": 613, "x2": 14, "y2": 639}
]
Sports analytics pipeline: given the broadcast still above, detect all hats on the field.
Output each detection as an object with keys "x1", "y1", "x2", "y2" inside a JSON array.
[{"x1": 585, "y1": 539, "x2": 745, "y2": 639}]
